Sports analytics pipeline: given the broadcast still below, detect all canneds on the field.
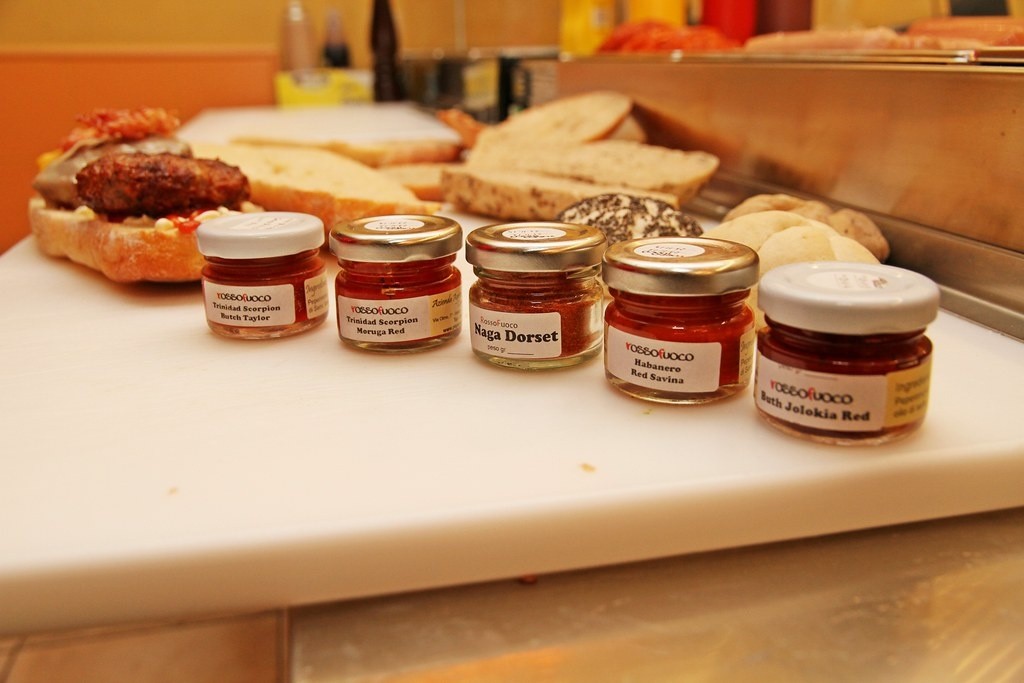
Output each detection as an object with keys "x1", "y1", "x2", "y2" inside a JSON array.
[
  {"x1": 602, "y1": 235, "x2": 761, "y2": 406},
  {"x1": 195, "y1": 212, "x2": 327, "y2": 339},
  {"x1": 329, "y1": 215, "x2": 463, "y2": 352},
  {"x1": 465, "y1": 219, "x2": 606, "y2": 370},
  {"x1": 756, "y1": 260, "x2": 938, "y2": 447}
]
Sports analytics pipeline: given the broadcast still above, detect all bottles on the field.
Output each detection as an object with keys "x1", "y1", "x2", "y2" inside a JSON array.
[{"x1": 275, "y1": 0, "x2": 404, "y2": 102}]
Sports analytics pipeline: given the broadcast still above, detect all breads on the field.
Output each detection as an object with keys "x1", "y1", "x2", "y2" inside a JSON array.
[
  {"x1": 743, "y1": 14, "x2": 1024, "y2": 51},
  {"x1": 30, "y1": 91, "x2": 892, "y2": 331}
]
